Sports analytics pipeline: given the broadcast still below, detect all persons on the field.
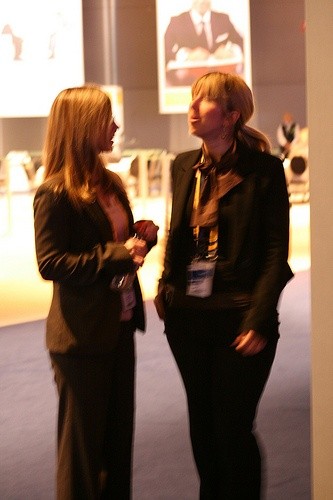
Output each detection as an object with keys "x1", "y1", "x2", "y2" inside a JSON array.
[
  {"x1": 276, "y1": 110, "x2": 300, "y2": 158},
  {"x1": 164, "y1": 0, "x2": 244, "y2": 59},
  {"x1": 32, "y1": 87, "x2": 158, "y2": 499},
  {"x1": 153, "y1": 70, "x2": 296, "y2": 500}
]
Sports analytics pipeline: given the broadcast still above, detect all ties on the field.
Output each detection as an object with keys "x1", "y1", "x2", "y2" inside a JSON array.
[{"x1": 193, "y1": 154, "x2": 218, "y2": 210}]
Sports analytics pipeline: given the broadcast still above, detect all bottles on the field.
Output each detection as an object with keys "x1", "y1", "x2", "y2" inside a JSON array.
[{"x1": 110, "y1": 226, "x2": 145, "y2": 292}]
